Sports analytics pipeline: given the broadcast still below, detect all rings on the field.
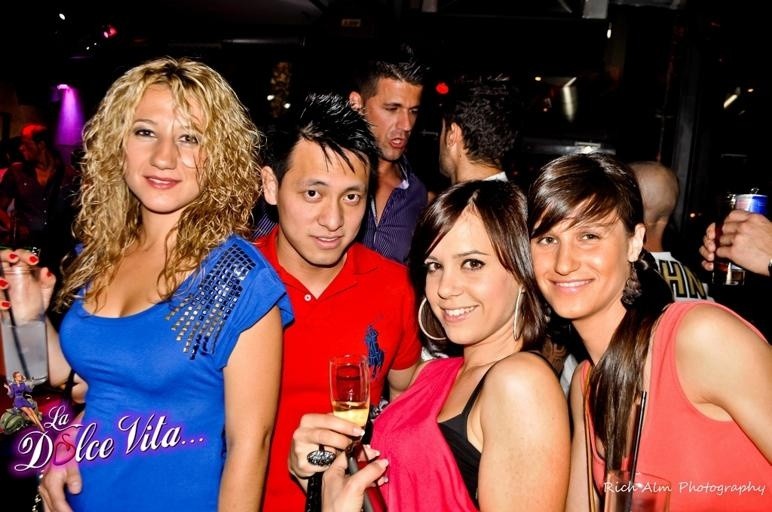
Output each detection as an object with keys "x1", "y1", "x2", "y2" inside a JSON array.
[{"x1": 307, "y1": 444, "x2": 337, "y2": 467}]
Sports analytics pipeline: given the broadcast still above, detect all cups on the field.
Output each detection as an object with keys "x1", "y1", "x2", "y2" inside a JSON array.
[
  {"x1": 329, "y1": 355, "x2": 371, "y2": 430},
  {"x1": 711, "y1": 190, "x2": 749, "y2": 288},
  {"x1": 0, "y1": 266, "x2": 49, "y2": 386}
]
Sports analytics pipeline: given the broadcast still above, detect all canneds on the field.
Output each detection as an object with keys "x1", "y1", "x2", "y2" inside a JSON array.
[{"x1": 734, "y1": 187, "x2": 768, "y2": 216}]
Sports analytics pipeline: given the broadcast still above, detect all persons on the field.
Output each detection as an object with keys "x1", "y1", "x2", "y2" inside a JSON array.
[
  {"x1": 526, "y1": 153, "x2": 771, "y2": 512},
  {"x1": 33, "y1": 89, "x2": 422, "y2": 510},
  {"x1": 242, "y1": 36, "x2": 435, "y2": 272},
  {"x1": 1, "y1": 120, "x2": 87, "y2": 334},
  {"x1": 286, "y1": 180, "x2": 572, "y2": 510},
  {"x1": 420, "y1": 64, "x2": 582, "y2": 400},
  {"x1": 697, "y1": 204, "x2": 771, "y2": 287},
  {"x1": 630, "y1": 159, "x2": 714, "y2": 303},
  {"x1": 2, "y1": 59, "x2": 296, "y2": 510},
  {"x1": 4, "y1": 371, "x2": 48, "y2": 434}
]
